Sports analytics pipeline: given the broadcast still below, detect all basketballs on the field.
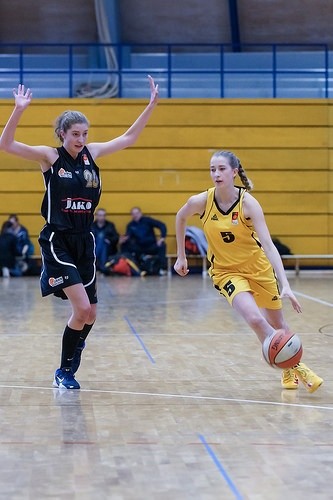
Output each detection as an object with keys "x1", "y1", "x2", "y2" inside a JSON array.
[{"x1": 262, "y1": 329, "x2": 303, "y2": 370}]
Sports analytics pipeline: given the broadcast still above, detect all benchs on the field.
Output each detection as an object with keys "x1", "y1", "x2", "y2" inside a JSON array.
[{"x1": 28, "y1": 254, "x2": 209, "y2": 277}]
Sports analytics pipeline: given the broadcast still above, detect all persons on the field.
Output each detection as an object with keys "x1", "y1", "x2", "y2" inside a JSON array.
[
  {"x1": 122, "y1": 208, "x2": 169, "y2": 276},
  {"x1": 91, "y1": 208, "x2": 121, "y2": 276},
  {"x1": 174, "y1": 150, "x2": 323, "y2": 393},
  {"x1": 0, "y1": 221, "x2": 23, "y2": 278},
  {"x1": 7, "y1": 214, "x2": 35, "y2": 275},
  {"x1": 0, "y1": 75, "x2": 159, "y2": 389}
]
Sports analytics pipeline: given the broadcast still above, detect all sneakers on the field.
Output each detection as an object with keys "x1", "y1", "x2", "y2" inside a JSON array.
[
  {"x1": 280, "y1": 369, "x2": 298, "y2": 389},
  {"x1": 53, "y1": 366, "x2": 81, "y2": 389},
  {"x1": 292, "y1": 363, "x2": 323, "y2": 393}
]
